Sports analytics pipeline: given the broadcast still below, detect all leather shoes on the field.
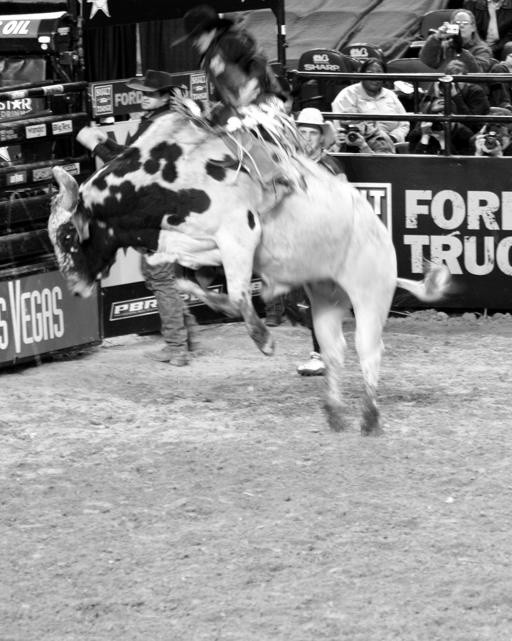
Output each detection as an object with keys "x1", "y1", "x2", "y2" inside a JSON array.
[{"x1": 143, "y1": 347, "x2": 189, "y2": 367}]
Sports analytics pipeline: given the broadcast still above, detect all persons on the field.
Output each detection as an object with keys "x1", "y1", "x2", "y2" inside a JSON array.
[
  {"x1": 329, "y1": 108, "x2": 395, "y2": 152},
  {"x1": 410, "y1": 0, "x2": 512, "y2": 157},
  {"x1": 293, "y1": 107, "x2": 346, "y2": 374},
  {"x1": 77, "y1": 70, "x2": 199, "y2": 365},
  {"x1": 331, "y1": 60, "x2": 411, "y2": 154},
  {"x1": 168, "y1": 8, "x2": 293, "y2": 145}
]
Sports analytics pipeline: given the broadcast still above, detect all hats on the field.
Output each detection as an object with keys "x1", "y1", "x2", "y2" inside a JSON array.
[
  {"x1": 291, "y1": 108, "x2": 336, "y2": 149},
  {"x1": 127, "y1": 70, "x2": 188, "y2": 93},
  {"x1": 170, "y1": 5, "x2": 234, "y2": 47}
]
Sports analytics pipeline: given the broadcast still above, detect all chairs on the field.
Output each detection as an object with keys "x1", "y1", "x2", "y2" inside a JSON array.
[{"x1": 270, "y1": 9, "x2": 512, "y2": 154}]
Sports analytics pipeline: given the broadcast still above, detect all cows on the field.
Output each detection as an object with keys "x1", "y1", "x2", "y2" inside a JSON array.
[{"x1": 48, "y1": 111, "x2": 451, "y2": 437}]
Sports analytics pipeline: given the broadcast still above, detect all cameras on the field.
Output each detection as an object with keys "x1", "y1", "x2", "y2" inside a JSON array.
[
  {"x1": 336, "y1": 123, "x2": 360, "y2": 142},
  {"x1": 483, "y1": 131, "x2": 504, "y2": 150},
  {"x1": 446, "y1": 24, "x2": 460, "y2": 35}
]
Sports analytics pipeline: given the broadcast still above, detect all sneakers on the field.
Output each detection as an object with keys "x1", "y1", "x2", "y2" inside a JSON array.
[
  {"x1": 298, "y1": 350, "x2": 325, "y2": 376},
  {"x1": 264, "y1": 313, "x2": 282, "y2": 327}
]
